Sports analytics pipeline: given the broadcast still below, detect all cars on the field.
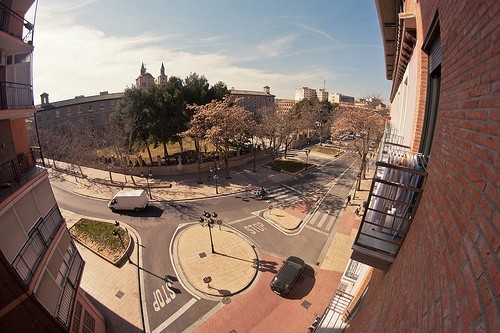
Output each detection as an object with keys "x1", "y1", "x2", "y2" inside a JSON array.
[{"x1": 330, "y1": 132, "x2": 360, "y2": 144}]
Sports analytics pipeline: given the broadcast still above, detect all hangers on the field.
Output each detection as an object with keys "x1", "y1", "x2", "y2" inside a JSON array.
[{"x1": 388, "y1": 146, "x2": 428, "y2": 170}]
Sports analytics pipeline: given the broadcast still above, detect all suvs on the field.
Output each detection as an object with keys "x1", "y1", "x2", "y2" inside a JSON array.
[{"x1": 270, "y1": 255, "x2": 305, "y2": 298}]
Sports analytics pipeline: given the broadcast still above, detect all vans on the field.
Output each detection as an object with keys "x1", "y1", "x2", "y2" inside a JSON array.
[{"x1": 108, "y1": 189, "x2": 148, "y2": 212}]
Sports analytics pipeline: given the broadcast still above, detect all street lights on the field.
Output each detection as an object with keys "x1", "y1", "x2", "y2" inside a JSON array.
[
  {"x1": 197, "y1": 211, "x2": 217, "y2": 253},
  {"x1": 112, "y1": 221, "x2": 132, "y2": 263},
  {"x1": 304, "y1": 150, "x2": 311, "y2": 170},
  {"x1": 209, "y1": 164, "x2": 221, "y2": 194},
  {"x1": 140, "y1": 171, "x2": 153, "y2": 199},
  {"x1": 352, "y1": 174, "x2": 361, "y2": 200}
]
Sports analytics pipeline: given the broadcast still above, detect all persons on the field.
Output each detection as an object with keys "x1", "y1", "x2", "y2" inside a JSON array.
[
  {"x1": 258, "y1": 186, "x2": 268, "y2": 198},
  {"x1": 346, "y1": 194, "x2": 367, "y2": 217}
]
